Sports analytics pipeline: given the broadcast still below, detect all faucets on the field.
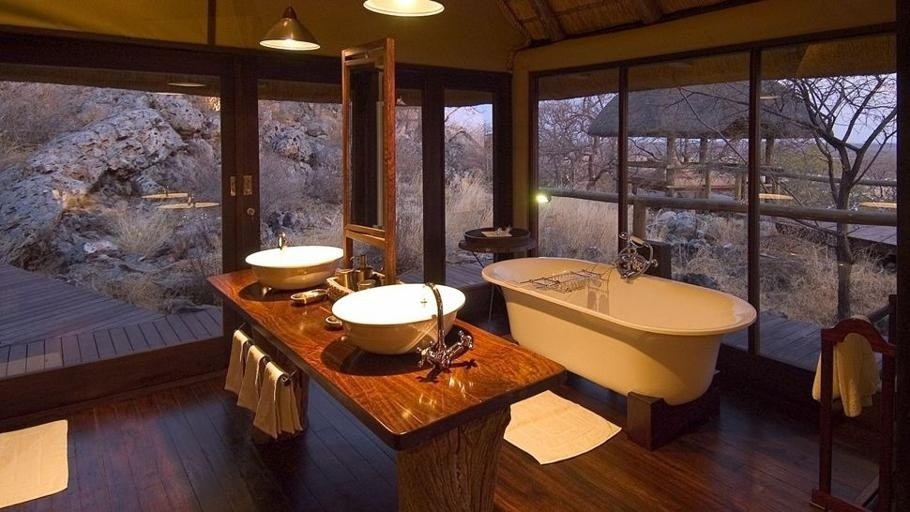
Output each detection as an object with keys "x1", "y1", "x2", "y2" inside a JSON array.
[
  {"x1": 278, "y1": 231, "x2": 287, "y2": 249},
  {"x1": 420, "y1": 280, "x2": 448, "y2": 352},
  {"x1": 620, "y1": 269, "x2": 635, "y2": 279}
]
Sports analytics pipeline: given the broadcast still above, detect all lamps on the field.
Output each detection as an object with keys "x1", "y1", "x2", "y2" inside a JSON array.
[{"x1": 256, "y1": 1, "x2": 446, "y2": 52}]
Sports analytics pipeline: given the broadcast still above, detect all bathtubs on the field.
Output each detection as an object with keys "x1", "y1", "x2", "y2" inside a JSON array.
[{"x1": 482, "y1": 256, "x2": 758, "y2": 408}]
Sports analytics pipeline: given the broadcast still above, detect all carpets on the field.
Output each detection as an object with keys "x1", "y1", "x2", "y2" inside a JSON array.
[
  {"x1": 1, "y1": 416, "x2": 73, "y2": 508},
  {"x1": 504, "y1": 389, "x2": 623, "y2": 466}
]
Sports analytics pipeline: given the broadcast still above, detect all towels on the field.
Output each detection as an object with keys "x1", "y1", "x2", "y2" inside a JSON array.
[
  {"x1": 809, "y1": 312, "x2": 878, "y2": 418},
  {"x1": 223, "y1": 330, "x2": 308, "y2": 440}
]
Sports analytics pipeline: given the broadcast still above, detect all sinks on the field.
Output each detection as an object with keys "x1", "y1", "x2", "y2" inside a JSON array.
[
  {"x1": 245, "y1": 244, "x2": 344, "y2": 290},
  {"x1": 331, "y1": 284, "x2": 466, "y2": 357}
]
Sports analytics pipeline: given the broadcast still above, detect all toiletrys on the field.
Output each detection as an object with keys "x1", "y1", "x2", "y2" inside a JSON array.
[{"x1": 347, "y1": 255, "x2": 373, "y2": 292}]
